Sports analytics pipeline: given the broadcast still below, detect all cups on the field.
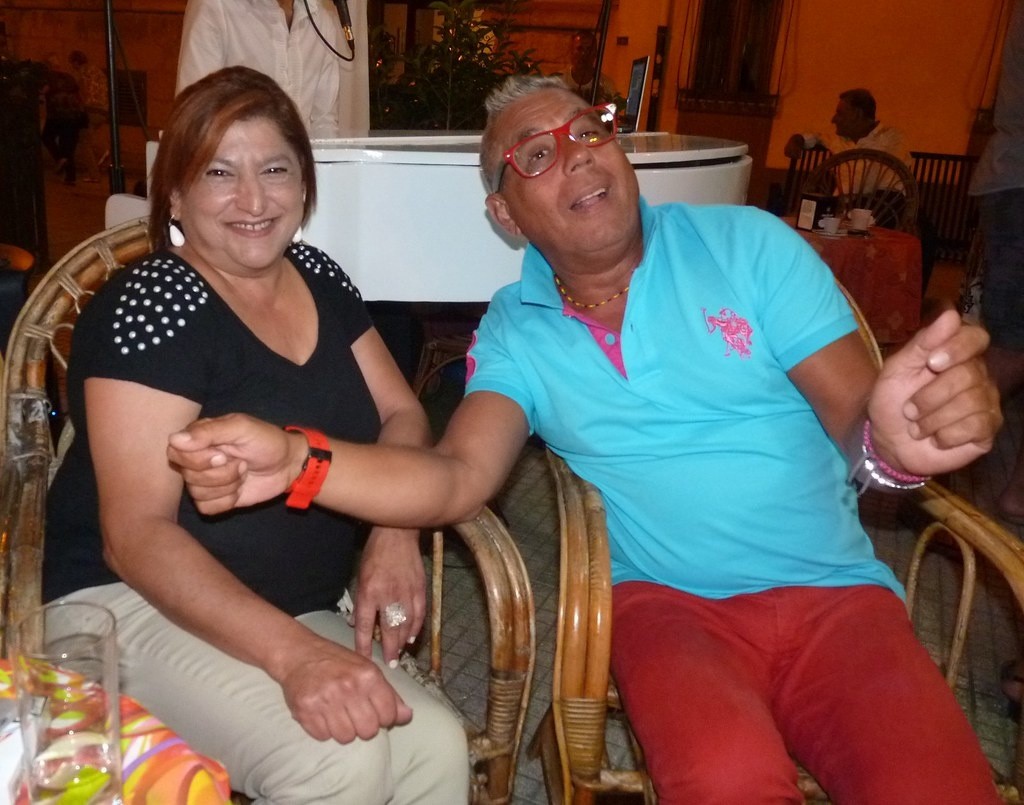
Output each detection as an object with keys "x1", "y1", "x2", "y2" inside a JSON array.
[
  {"x1": 818, "y1": 217, "x2": 841, "y2": 234},
  {"x1": 848, "y1": 209, "x2": 872, "y2": 231},
  {"x1": 7, "y1": 601, "x2": 124, "y2": 804}
]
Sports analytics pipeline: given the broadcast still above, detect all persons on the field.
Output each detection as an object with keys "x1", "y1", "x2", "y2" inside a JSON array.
[
  {"x1": 808, "y1": 86, "x2": 913, "y2": 228},
  {"x1": 38, "y1": 66, "x2": 87, "y2": 184},
  {"x1": 164, "y1": 78, "x2": 1008, "y2": 805},
  {"x1": 66, "y1": 50, "x2": 110, "y2": 182},
  {"x1": 176, "y1": 0, "x2": 344, "y2": 140},
  {"x1": 41, "y1": 63, "x2": 470, "y2": 805},
  {"x1": 964, "y1": 0, "x2": 1023, "y2": 347}
]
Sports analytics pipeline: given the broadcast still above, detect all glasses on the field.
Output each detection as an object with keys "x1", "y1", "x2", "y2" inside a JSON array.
[{"x1": 493, "y1": 104, "x2": 617, "y2": 197}]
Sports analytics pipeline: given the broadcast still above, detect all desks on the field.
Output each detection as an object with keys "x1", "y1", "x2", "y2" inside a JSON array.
[{"x1": 779, "y1": 214, "x2": 923, "y2": 357}]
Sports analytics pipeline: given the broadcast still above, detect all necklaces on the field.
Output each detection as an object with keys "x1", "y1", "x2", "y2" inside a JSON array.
[{"x1": 553, "y1": 273, "x2": 631, "y2": 308}]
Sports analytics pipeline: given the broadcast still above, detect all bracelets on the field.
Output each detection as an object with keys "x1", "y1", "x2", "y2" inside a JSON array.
[
  {"x1": 283, "y1": 425, "x2": 332, "y2": 510},
  {"x1": 848, "y1": 420, "x2": 932, "y2": 499}
]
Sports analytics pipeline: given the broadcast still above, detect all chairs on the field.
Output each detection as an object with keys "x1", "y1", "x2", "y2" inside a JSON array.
[
  {"x1": 0, "y1": 216, "x2": 533, "y2": 805},
  {"x1": 793, "y1": 147, "x2": 918, "y2": 234},
  {"x1": 910, "y1": 150, "x2": 983, "y2": 284},
  {"x1": 524, "y1": 280, "x2": 1023, "y2": 805},
  {"x1": 779, "y1": 143, "x2": 837, "y2": 216}
]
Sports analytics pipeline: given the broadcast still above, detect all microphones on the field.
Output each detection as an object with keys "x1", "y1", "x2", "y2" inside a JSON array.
[{"x1": 333, "y1": 0, "x2": 356, "y2": 50}]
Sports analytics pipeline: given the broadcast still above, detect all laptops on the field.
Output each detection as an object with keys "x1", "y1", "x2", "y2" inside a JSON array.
[{"x1": 621, "y1": 55, "x2": 650, "y2": 132}]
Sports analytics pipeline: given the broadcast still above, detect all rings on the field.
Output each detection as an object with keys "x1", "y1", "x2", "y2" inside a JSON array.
[{"x1": 384, "y1": 602, "x2": 406, "y2": 631}]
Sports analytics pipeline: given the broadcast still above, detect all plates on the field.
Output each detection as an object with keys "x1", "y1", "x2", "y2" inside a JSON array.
[{"x1": 812, "y1": 228, "x2": 846, "y2": 240}]
